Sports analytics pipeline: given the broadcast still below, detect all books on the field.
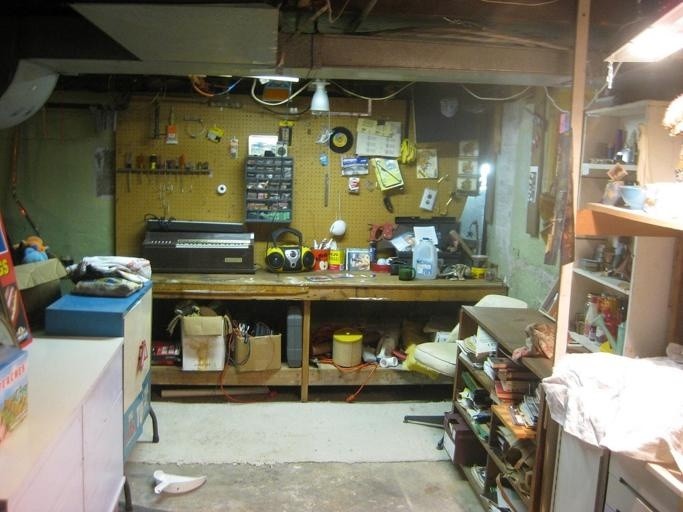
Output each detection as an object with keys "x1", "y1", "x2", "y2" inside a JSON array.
[{"x1": 454, "y1": 323, "x2": 542, "y2": 429}]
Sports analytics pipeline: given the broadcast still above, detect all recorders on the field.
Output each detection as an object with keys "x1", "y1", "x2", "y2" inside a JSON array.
[{"x1": 265, "y1": 227, "x2": 316, "y2": 274}]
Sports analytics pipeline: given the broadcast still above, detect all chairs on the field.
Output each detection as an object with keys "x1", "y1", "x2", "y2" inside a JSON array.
[{"x1": 398, "y1": 293, "x2": 531, "y2": 449}]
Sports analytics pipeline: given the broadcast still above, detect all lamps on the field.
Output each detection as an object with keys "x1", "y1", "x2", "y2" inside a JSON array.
[
  {"x1": 602, "y1": 0, "x2": 683, "y2": 64},
  {"x1": 310, "y1": 79, "x2": 330, "y2": 112}
]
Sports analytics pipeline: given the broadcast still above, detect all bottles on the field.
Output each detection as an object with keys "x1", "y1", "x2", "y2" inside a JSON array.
[{"x1": 583, "y1": 292, "x2": 600, "y2": 343}]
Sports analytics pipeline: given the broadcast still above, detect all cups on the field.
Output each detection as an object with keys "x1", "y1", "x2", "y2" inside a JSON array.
[{"x1": 398, "y1": 265, "x2": 416, "y2": 281}]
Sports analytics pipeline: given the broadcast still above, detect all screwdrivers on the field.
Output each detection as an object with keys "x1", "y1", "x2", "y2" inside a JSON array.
[{"x1": 127, "y1": 153, "x2": 196, "y2": 193}]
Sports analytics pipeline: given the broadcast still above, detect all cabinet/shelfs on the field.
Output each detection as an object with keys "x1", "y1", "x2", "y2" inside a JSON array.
[
  {"x1": 308, "y1": 269, "x2": 509, "y2": 385},
  {"x1": 563, "y1": 109, "x2": 670, "y2": 362},
  {"x1": 124, "y1": 282, "x2": 151, "y2": 467},
  {"x1": 10, "y1": 330, "x2": 122, "y2": 512},
  {"x1": 150, "y1": 271, "x2": 306, "y2": 394},
  {"x1": 447, "y1": 304, "x2": 561, "y2": 512}
]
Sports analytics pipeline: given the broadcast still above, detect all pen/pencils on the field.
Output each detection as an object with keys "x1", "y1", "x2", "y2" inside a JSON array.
[{"x1": 314, "y1": 238, "x2": 333, "y2": 250}]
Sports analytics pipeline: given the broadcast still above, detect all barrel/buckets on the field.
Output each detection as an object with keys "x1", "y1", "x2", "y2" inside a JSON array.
[{"x1": 413, "y1": 237, "x2": 438, "y2": 280}]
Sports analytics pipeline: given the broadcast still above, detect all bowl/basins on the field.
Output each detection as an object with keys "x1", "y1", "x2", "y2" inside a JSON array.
[{"x1": 616, "y1": 185, "x2": 646, "y2": 210}]
